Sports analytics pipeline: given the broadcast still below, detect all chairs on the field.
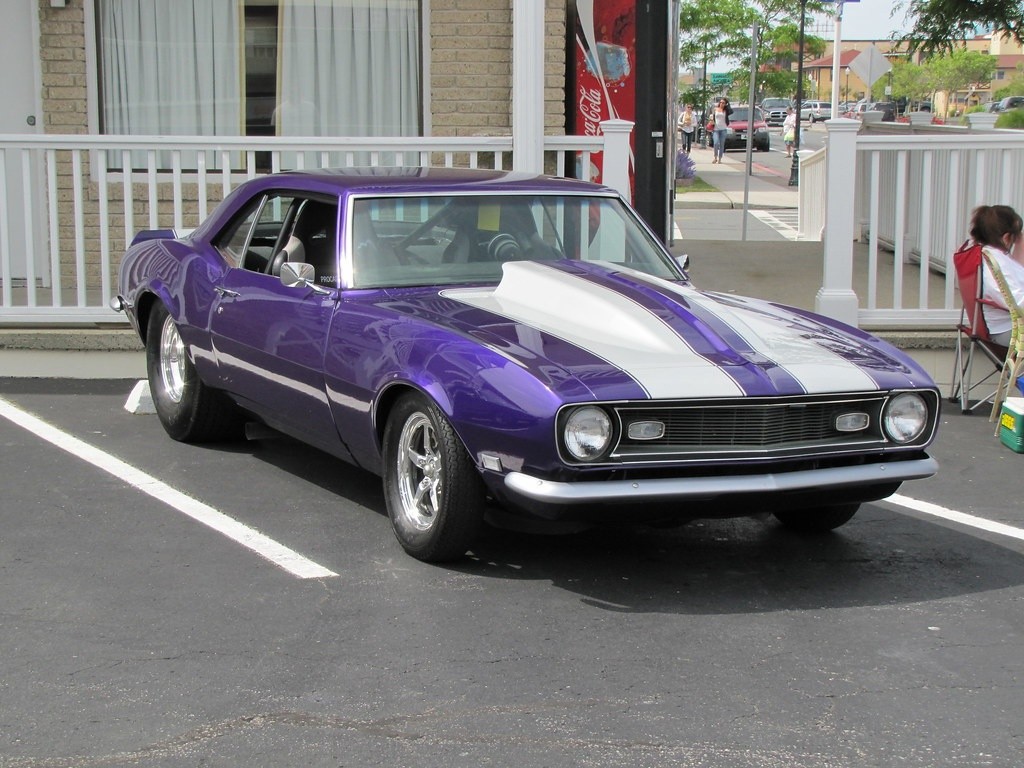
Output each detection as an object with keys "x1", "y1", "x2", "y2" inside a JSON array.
[
  {"x1": 441, "y1": 203, "x2": 565, "y2": 265},
  {"x1": 980, "y1": 248, "x2": 1024, "y2": 438},
  {"x1": 948, "y1": 239, "x2": 1023, "y2": 415},
  {"x1": 271, "y1": 198, "x2": 399, "y2": 279}
]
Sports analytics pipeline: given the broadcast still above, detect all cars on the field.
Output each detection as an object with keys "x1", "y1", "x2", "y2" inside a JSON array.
[
  {"x1": 759, "y1": 97, "x2": 794, "y2": 127},
  {"x1": 797, "y1": 98, "x2": 934, "y2": 125},
  {"x1": 108, "y1": 165, "x2": 942, "y2": 566},
  {"x1": 691, "y1": 112, "x2": 702, "y2": 141},
  {"x1": 983, "y1": 96, "x2": 1024, "y2": 113},
  {"x1": 704, "y1": 102, "x2": 770, "y2": 152}
]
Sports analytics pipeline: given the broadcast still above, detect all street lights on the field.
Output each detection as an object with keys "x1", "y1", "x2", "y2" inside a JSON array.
[{"x1": 845, "y1": 67, "x2": 850, "y2": 112}]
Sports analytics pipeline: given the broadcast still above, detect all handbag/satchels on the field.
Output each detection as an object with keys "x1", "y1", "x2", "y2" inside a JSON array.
[
  {"x1": 677, "y1": 125, "x2": 682, "y2": 131},
  {"x1": 706, "y1": 120, "x2": 714, "y2": 132},
  {"x1": 784, "y1": 127, "x2": 804, "y2": 145}
]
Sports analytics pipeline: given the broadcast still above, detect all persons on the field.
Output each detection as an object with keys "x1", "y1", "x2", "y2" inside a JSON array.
[
  {"x1": 970, "y1": 205, "x2": 1024, "y2": 349},
  {"x1": 710, "y1": 98, "x2": 733, "y2": 164},
  {"x1": 678, "y1": 105, "x2": 697, "y2": 158},
  {"x1": 780, "y1": 107, "x2": 796, "y2": 158}
]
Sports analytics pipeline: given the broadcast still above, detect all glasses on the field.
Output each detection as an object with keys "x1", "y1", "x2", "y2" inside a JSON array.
[
  {"x1": 687, "y1": 106, "x2": 692, "y2": 108},
  {"x1": 719, "y1": 101, "x2": 725, "y2": 104}
]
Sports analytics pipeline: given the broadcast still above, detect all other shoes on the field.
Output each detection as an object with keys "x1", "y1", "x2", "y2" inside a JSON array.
[
  {"x1": 718, "y1": 158, "x2": 721, "y2": 164},
  {"x1": 712, "y1": 158, "x2": 717, "y2": 163},
  {"x1": 786, "y1": 155, "x2": 791, "y2": 158}
]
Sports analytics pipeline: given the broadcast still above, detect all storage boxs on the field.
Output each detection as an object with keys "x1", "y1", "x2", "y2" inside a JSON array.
[{"x1": 1001, "y1": 396, "x2": 1024, "y2": 453}]
